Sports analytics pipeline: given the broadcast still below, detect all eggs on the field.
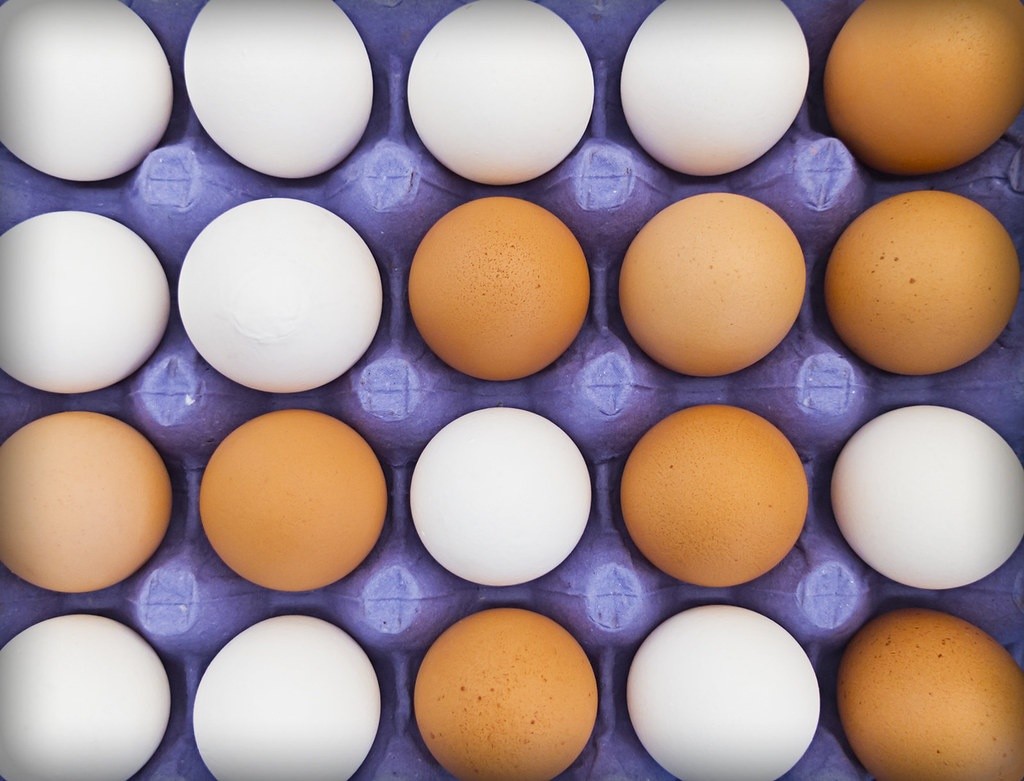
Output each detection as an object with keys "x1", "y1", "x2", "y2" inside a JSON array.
[{"x1": 0, "y1": 0, "x2": 1024, "y2": 781}]
